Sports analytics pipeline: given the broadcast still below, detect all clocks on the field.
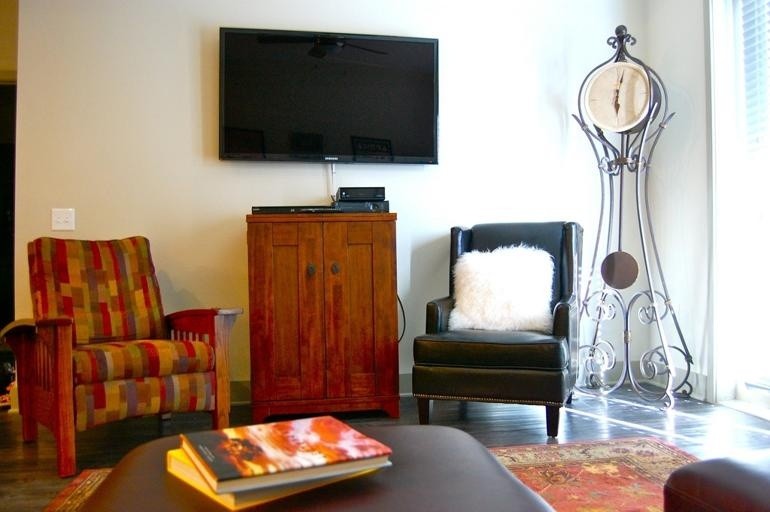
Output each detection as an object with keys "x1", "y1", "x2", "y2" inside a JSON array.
[{"x1": 556, "y1": 26, "x2": 695, "y2": 406}]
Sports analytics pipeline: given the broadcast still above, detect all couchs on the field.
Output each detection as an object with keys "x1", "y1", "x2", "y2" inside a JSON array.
[{"x1": 662, "y1": 447, "x2": 769, "y2": 512}]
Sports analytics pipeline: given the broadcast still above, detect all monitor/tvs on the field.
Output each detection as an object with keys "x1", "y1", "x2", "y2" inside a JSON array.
[{"x1": 217, "y1": 25, "x2": 442, "y2": 167}]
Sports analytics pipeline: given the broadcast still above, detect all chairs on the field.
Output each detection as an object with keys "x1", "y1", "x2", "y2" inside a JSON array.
[
  {"x1": 0, "y1": 236, "x2": 244, "y2": 478},
  {"x1": 411, "y1": 222, "x2": 584, "y2": 439}
]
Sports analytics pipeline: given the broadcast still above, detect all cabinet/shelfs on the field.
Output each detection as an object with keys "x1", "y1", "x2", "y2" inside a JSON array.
[{"x1": 246, "y1": 214, "x2": 400, "y2": 427}]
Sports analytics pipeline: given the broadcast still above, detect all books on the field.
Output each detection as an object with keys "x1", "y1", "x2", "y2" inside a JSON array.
[
  {"x1": 179, "y1": 415, "x2": 393, "y2": 495},
  {"x1": 165, "y1": 447, "x2": 376, "y2": 512}
]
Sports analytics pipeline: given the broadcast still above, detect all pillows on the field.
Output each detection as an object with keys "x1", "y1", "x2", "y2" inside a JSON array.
[{"x1": 447, "y1": 244, "x2": 556, "y2": 336}]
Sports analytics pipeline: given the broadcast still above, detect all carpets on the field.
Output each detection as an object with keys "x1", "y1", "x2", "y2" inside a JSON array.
[{"x1": 42, "y1": 434, "x2": 703, "y2": 512}]
